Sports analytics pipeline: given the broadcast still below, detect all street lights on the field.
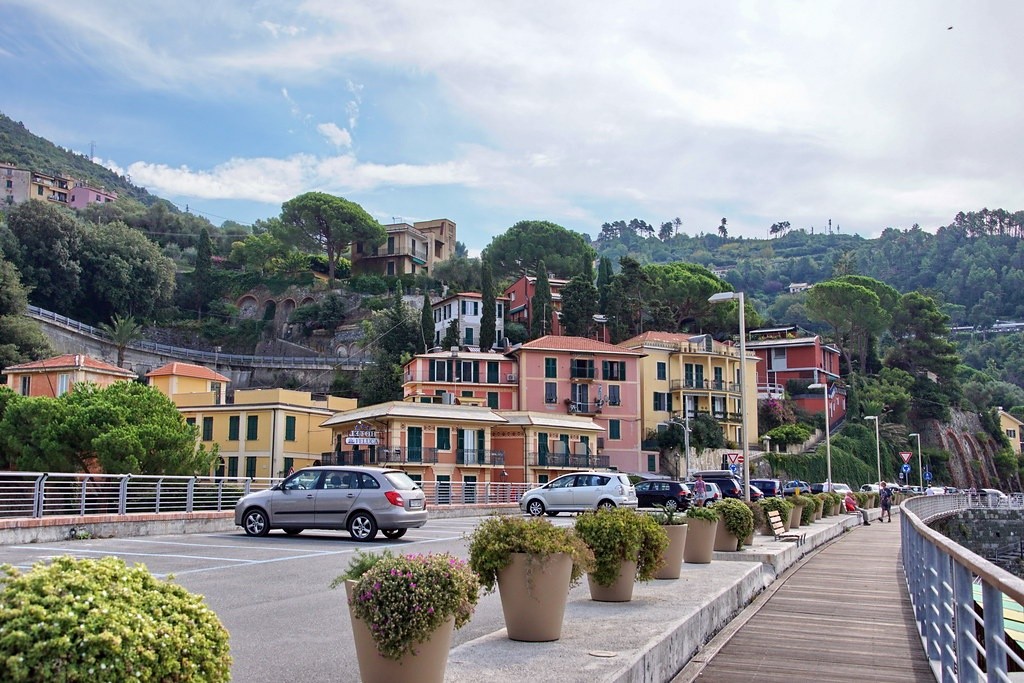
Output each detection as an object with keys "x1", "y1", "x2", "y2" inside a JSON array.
[
  {"x1": 500, "y1": 470, "x2": 509, "y2": 503},
  {"x1": 674, "y1": 452, "x2": 680, "y2": 481},
  {"x1": 909, "y1": 433, "x2": 923, "y2": 494},
  {"x1": 808, "y1": 383, "x2": 832, "y2": 494},
  {"x1": 708, "y1": 293, "x2": 750, "y2": 502},
  {"x1": 662, "y1": 421, "x2": 689, "y2": 479},
  {"x1": 864, "y1": 416, "x2": 881, "y2": 490}
]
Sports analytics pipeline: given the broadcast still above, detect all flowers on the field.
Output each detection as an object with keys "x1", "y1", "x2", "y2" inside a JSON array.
[{"x1": 328, "y1": 547, "x2": 479, "y2": 658}]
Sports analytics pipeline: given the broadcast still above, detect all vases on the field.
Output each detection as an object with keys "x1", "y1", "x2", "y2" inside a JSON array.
[{"x1": 343, "y1": 579, "x2": 456, "y2": 683}]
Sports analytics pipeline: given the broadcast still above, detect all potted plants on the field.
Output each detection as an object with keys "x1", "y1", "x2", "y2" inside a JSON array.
[
  {"x1": 652, "y1": 508, "x2": 688, "y2": 580},
  {"x1": 846, "y1": 490, "x2": 907, "y2": 509},
  {"x1": 684, "y1": 504, "x2": 720, "y2": 565},
  {"x1": 743, "y1": 502, "x2": 761, "y2": 545},
  {"x1": 574, "y1": 507, "x2": 667, "y2": 604},
  {"x1": 465, "y1": 515, "x2": 594, "y2": 644},
  {"x1": 710, "y1": 498, "x2": 753, "y2": 552},
  {"x1": 761, "y1": 488, "x2": 842, "y2": 536}
]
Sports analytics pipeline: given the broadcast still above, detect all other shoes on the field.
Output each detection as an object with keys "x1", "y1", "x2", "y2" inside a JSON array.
[
  {"x1": 878, "y1": 517, "x2": 883, "y2": 522},
  {"x1": 863, "y1": 521, "x2": 870, "y2": 526},
  {"x1": 888, "y1": 518, "x2": 891, "y2": 522}
]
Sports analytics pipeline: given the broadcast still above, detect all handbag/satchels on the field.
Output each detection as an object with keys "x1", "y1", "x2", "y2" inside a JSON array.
[{"x1": 845, "y1": 502, "x2": 855, "y2": 511}]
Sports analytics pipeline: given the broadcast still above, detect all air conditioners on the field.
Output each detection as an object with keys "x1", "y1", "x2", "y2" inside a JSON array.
[{"x1": 506, "y1": 373, "x2": 515, "y2": 381}]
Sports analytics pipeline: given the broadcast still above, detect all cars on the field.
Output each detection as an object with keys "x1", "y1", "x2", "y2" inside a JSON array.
[
  {"x1": 749, "y1": 479, "x2": 784, "y2": 498},
  {"x1": 633, "y1": 480, "x2": 692, "y2": 512},
  {"x1": 782, "y1": 481, "x2": 811, "y2": 496},
  {"x1": 875, "y1": 482, "x2": 1014, "y2": 503},
  {"x1": 832, "y1": 483, "x2": 852, "y2": 494},
  {"x1": 235, "y1": 465, "x2": 429, "y2": 541},
  {"x1": 686, "y1": 482, "x2": 722, "y2": 508},
  {"x1": 519, "y1": 472, "x2": 639, "y2": 517},
  {"x1": 741, "y1": 483, "x2": 764, "y2": 502},
  {"x1": 859, "y1": 484, "x2": 884, "y2": 492},
  {"x1": 811, "y1": 483, "x2": 825, "y2": 495},
  {"x1": 693, "y1": 470, "x2": 741, "y2": 499}
]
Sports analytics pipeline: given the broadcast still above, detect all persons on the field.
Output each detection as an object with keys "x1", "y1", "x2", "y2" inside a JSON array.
[
  {"x1": 585, "y1": 470, "x2": 600, "y2": 486},
  {"x1": 693, "y1": 474, "x2": 707, "y2": 508},
  {"x1": 303, "y1": 460, "x2": 321, "y2": 477},
  {"x1": 925, "y1": 486, "x2": 934, "y2": 495},
  {"x1": 845, "y1": 492, "x2": 871, "y2": 526},
  {"x1": 944, "y1": 486, "x2": 947, "y2": 493},
  {"x1": 823, "y1": 478, "x2": 834, "y2": 492},
  {"x1": 897, "y1": 480, "x2": 903, "y2": 487},
  {"x1": 878, "y1": 481, "x2": 892, "y2": 522},
  {"x1": 970, "y1": 485, "x2": 977, "y2": 496}
]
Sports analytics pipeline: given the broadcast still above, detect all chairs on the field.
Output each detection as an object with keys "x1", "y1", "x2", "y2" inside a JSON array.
[
  {"x1": 364, "y1": 479, "x2": 374, "y2": 486},
  {"x1": 343, "y1": 476, "x2": 351, "y2": 488},
  {"x1": 579, "y1": 479, "x2": 584, "y2": 486},
  {"x1": 331, "y1": 476, "x2": 340, "y2": 489}
]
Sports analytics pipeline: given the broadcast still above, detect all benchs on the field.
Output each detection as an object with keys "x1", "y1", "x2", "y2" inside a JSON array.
[
  {"x1": 767, "y1": 511, "x2": 807, "y2": 548},
  {"x1": 841, "y1": 501, "x2": 860, "y2": 516}
]
